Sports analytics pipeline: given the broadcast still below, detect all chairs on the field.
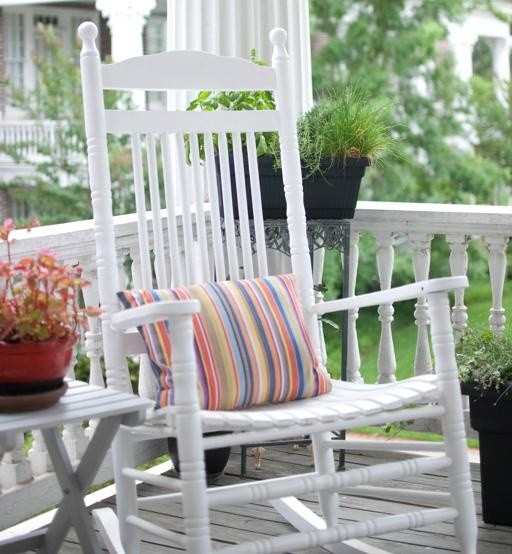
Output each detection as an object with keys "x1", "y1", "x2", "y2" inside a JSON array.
[{"x1": 77, "y1": 20, "x2": 478, "y2": 554}]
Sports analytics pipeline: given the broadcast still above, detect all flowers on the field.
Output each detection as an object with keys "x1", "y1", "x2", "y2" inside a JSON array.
[{"x1": 0, "y1": 213, "x2": 109, "y2": 342}]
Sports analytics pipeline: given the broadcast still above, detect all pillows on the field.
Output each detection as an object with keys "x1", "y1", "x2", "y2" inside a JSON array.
[{"x1": 116, "y1": 275, "x2": 333, "y2": 408}]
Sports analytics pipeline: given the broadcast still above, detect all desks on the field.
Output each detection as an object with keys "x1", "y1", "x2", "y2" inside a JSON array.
[{"x1": 0, "y1": 376, "x2": 154, "y2": 554}]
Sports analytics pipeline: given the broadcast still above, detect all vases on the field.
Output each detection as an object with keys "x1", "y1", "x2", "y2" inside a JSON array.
[{"x1": 0, "y1": 341, "x2": 76, "y2": 413}]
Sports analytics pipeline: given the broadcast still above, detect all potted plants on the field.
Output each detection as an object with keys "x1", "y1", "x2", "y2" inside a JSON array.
[
  {"x1": 182, "y1": 50, "x2": 421, "y2": 220},
  {"x1": 455, "y1": 320, "x2": 512, "y2": 528}
]
